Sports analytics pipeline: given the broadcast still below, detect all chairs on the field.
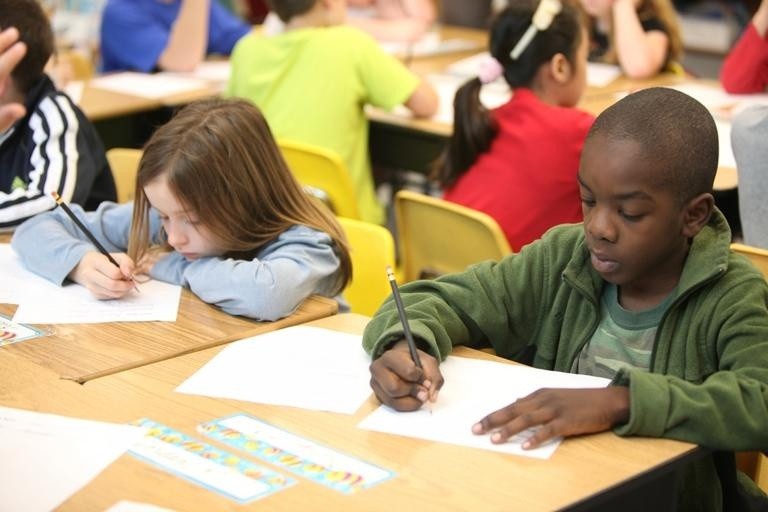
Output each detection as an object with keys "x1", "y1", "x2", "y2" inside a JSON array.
[
  {"x1": 327, "y1": 216, "x2": 395, "y2": 320},
  {"x1": 390, "y1": 191, "x2": 519, "y2": 349},
  {"x1": 277, "y1": 143, "x2": 357, "y2": 220},
  {"x1": 730, "y1": 240, "x2": 768, "y2": 490},
  {"x1": 106, "y1": 148, "x2": 146, "y2": 208}
]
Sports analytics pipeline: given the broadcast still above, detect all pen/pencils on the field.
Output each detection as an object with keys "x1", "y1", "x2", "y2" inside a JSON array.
[
  {"x1": 52, "y1": 192, "x2": 140, "y2": 292},
  {"x1": 387, "y1": 267, "x2": 437, "y2": 404}
]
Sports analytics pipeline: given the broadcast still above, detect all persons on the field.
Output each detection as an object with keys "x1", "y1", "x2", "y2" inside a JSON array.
[
  {"x1": 426, "y1": 0, "x2": 767, "y2": 253},
  {"x1": 1, "y1": 1, "x2": 439, "y2": 323},
  {"x1": 363, "y1": 86, "x2": 767, "y2": 512}
]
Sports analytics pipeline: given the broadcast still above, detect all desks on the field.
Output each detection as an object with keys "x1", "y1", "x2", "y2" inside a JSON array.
[
  {"x1": 0, "y1": 207, "x2": 700, "y2": 511},
  {"x1": 2, "y1": 1, "x2": 768, "y2": 193}
]
